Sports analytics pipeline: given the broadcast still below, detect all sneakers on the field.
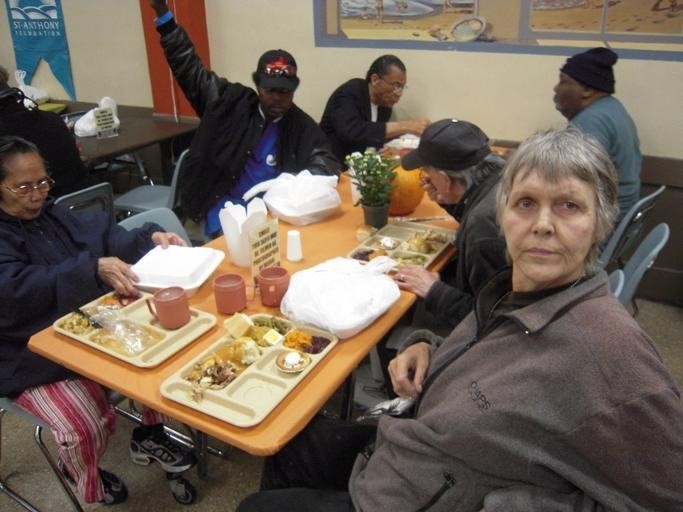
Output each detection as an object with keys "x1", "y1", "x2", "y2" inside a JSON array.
[
  {"x1": 58, "y1": 459, "x2": 128, "y2": 504},
  {"x1": 129, "y1": 423, "x2": 197, "y2": 473}
]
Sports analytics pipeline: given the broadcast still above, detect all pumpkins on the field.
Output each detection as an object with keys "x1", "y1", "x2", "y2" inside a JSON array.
[{"x1": 387, "y1": 164, "x2": 426, "y2": 217}]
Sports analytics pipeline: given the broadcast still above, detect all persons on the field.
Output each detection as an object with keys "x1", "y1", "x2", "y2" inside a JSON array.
[
  {"x1": 149, "y1": 0, "x2": 342, "y2": 241},
  {"x1": 317, "y1": 53, "x2": 431, "y2": 173},
  {"x1": 552, "y1": 47, "x2": 642, "y2": 258},
  {"x1": 233, "y1": 122, "x2": 683, "y2": 511},
  {"x1": 0, "y1": 66, "x2": 97, "y2": 201},
  {"x1": 0, "y1": 135, "x2": 196, "y2": 506},
  {"x1": 374, "y1": 116, "x2": 511, "y2": 400}
]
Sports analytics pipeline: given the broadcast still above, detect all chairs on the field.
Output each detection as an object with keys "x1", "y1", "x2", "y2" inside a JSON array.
[
  {"x1": 54, "y1": 183, "x2": 117, "y2": 225},
  {"x1": 115, "y1": 206, "x2": 191, "y2": 245},
  {"x1": 617, "y1": 220, "x2": 671, "y2": 306},
  {"x1": 0, "y1": 386, "x2": 225, "y2": 512},
  {"x1": 112, "y1": 148, "x2": 192, "y2": 214},
  {"x1": 600, "y1": 184, "x2": 669, "y2": 266}
]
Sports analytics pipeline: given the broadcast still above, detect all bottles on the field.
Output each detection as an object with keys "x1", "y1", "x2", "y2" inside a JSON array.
[{"x1": 287, "y1": 230, "x2": 302, "y2": 261}]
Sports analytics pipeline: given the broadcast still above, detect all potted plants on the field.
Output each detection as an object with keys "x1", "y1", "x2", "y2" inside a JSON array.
[{"x1": 344, "y1": 148, "x2": 404, "y2": 228}]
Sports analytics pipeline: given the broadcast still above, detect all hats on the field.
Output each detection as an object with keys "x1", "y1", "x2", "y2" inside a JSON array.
[
  {"x1": 400, "y1": 118, "x2": 490, "y2": 170},
  {"x1": 256, "y1": 48, "x2": 299, "y2": 91},
  {"x1": 560, "y1": 47, "x2": 617, "y2": 94}
]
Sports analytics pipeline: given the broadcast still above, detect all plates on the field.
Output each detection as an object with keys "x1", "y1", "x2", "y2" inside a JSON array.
[
  {"x1": 277, "y1": 349, "x2": 310, "y2": 374},
  {"x1": 131, "y1": 244, "x2": 224, "y2": 300}
]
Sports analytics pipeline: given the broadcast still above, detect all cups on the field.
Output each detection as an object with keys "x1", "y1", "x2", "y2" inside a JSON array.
[
  {"x1": 146, "y1": 286, "x2": 190, "y2": 330},
  {"x1": 260, "y1": 266, "x2": 289, "y2": 307},
  {"x1": 213, "y1": 274, "x2": 247, "y2": 314}
]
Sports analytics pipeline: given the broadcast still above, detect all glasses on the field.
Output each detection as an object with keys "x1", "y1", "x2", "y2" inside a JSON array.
[{"x1": 4, "y1": 177, "x2": 55, "y2": 196}]
